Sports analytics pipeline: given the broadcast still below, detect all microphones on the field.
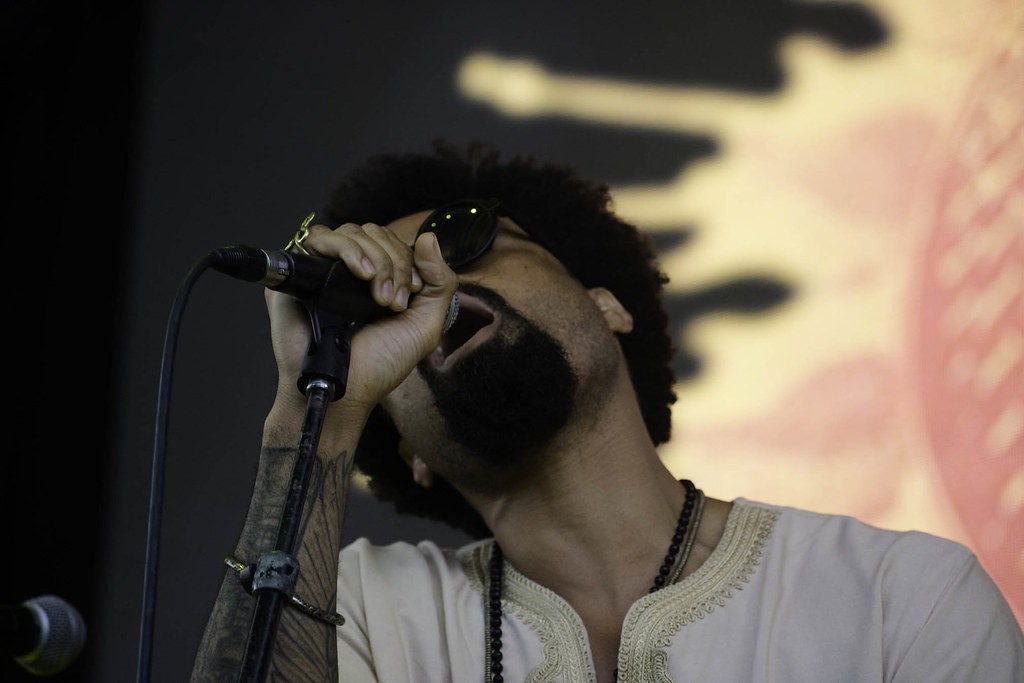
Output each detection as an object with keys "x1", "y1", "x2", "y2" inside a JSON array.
[
  {"x1": 212, "y1": 245, "x2": 460, "y2": 334},
  {"x1": 0, "y1": 596, "x2": 87, "y2": 676}
]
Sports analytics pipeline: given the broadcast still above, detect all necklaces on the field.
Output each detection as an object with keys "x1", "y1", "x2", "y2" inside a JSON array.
[{"x1": 485, "y1": 480, "x2": 706, "y2": 683}]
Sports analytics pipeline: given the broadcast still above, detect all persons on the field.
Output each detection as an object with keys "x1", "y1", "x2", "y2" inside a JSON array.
[{"x1": 189, "y1": 140, "x2": 1024, "y2": 683}]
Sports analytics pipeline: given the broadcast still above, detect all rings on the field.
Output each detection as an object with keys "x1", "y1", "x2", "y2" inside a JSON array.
[{"x1": 283, "y1": 212, "x2": 316, "y2": 255}]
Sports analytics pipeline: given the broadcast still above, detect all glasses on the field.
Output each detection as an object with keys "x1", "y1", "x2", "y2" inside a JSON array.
[{"x1": 409, "y1": 198, "x2": 555, "y2": 270}]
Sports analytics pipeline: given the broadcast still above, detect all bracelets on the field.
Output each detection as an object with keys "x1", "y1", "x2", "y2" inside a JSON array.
[{"x1": 223, "y1": 556, "x2": 344, "y2": 626}]
